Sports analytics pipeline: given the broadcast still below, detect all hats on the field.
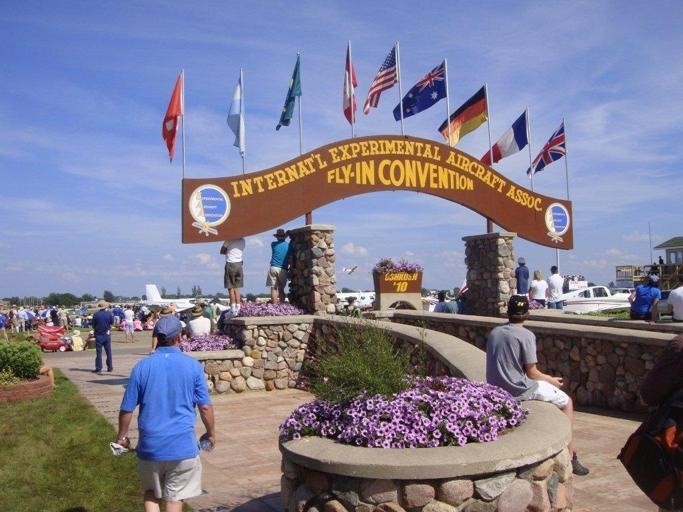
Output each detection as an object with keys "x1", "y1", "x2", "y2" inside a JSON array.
[
  {"x1": 96, "y1": 300, "x2": 133, "y2": 310},
  {"x1": 274, "y1": 229, "x2": 290, "y2": 240},
  {"x1": 159, "y1": 309, "x2": 173, "y2": 317},
  {"x1": 345, "y1": 296, "x2": 357, "y2": 300},
  {"x1": 191, "y1": 305, "x2": 203, "y2": 316},
  {"x1": 71, "y1": 329, "x2": 80, "y2": 337},
  {"x1": 507, "y1": 295, "x2": 529, "y2": 320},
  {"x1": 517, "y1": 257, "x2": 526, "y2": 264},
  {"x1": 151, "y1": 315, "x2": 182, "y2": 340},
  {"x1": 88, "y1": 330, "x2": 94, "y2": 337}
]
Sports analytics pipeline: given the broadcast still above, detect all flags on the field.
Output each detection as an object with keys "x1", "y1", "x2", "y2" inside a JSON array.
[
  {"x1": 527, "y1": 122, "x2": 567, "y2": 177},
  {"x1": 438, "y1": 86, "x2": 487, "y2": 148},
  {"x1": 393, "y1": 62, "x2": 446, "y2": 121},
  {"x1": 276, "y1": 55, "x2": 302, "y2": 131},
  {"x1": 343, "y1": 45, "x2": 359, "y2": 126},
  {"x1": 363, "y1": 44, "x2": 399, "y2": 115},
  {"x1": 227, "y1": 72, "x2": 246, "y2": 158},
  {"x1": 480, "y1": 109, "x2": 530, "y2": 166},
  {"x1": 162, "y1": 71, "x2": 183, "y2": 164}
]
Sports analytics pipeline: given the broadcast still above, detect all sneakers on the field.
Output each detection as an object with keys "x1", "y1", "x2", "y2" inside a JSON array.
[
  {"x1": 92, "y1": 367, "x2": 113, "y2": 373},
  {"x1": 224, "y1": 310, "x2": 240, "y2": 319},
  {"x1": 567, "y1": 451, "x2": 589, "y2": 478}
]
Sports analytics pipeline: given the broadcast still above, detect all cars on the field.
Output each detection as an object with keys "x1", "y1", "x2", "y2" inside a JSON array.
[
  {"x1": 657, "y1": 289, "x2": 671, "y2": 315},
  {"x1": 610, "y1": 287, "x2": 635, "y2": 299}
]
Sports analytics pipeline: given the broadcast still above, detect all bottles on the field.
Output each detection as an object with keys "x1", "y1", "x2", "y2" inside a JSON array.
[{"x1": 198, "y1": 434, "x2": 213, "y2": 452}]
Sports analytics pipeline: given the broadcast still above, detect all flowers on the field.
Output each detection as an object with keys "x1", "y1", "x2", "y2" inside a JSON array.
[
  {"x1": 177, "y1": 330, "x2": 237, "y2": 352},
  {"x1": 371, "y1": 257, "x2": 423, "y2": 274},
  {"x1": 275, "y1": 371, "x2": 530, "y2": 454},
  {"x1": 237, "y1": 299, "x2": 309, "y2": 319}
]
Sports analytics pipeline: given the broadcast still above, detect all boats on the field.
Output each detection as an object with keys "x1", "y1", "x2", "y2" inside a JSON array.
[{"x1": 559, "y1": 285, "x2": 632, "y2": 315}]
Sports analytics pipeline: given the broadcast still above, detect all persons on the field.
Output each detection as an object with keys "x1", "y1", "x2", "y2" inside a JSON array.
[
  {"x1": 515, "y1": 256, "x2": 585, "y2": 309},
  {"x1": 337, "y1": 297, "x2": 362, "y2": 319},
  {"x1": 220, "y1": 239, "x2": 245, "y2": 317},
  {"x1": 431, "y1": 289, "x2": 467, "y2": 314},
  {"x1": 629, "y1": 255, "x2": 683, "y2": 327},
  {"x1": 639, "y1": 335, "x2": 683, "y2": 512},
  {"x1": 0, "y1": 299, "x2": 230, "y2": 372},
  {"x1": 117, "y1": 315, "x2": 215, "y2": 512},
  {"x1": 265, "y1": 230, "x2": 289, "y2": 304},
  {"x1": 486, "y1": 294, "x2": 590, "y2": 475}
]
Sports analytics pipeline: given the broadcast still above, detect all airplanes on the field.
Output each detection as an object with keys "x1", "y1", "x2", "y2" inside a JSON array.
[{"x1": 137, "y1": 284, "x2": 375, "y2": 325}]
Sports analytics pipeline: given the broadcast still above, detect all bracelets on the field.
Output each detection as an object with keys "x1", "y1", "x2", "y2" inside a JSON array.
[{"x1": 117, "y1": 437, "x2": 128, "y2": 440}]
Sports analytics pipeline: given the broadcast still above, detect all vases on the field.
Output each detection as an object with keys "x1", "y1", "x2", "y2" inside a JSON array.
[{"x1": 372, "y1": 270, "x2": 421, "y2": 293}]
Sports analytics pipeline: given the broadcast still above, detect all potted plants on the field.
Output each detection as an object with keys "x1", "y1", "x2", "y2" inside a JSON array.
[{"x1": 0, "y1": 332, "x2": 51, "y2": 406}]
